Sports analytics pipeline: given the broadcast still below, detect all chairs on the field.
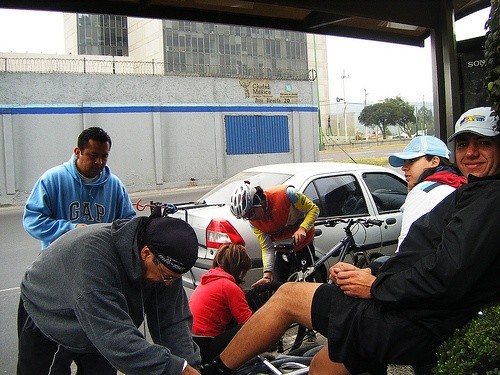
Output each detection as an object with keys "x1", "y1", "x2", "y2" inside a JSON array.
[{"x1": 325, "y1": 184, "x2": 350, "y2": 205}]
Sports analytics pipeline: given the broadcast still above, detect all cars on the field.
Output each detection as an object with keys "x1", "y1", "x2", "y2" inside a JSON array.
[{"x1": 158, "y1": 162, "x2": 409, "y2": 305}]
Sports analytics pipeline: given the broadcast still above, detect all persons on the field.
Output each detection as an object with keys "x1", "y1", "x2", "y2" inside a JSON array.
[
  {"x1": 388, "y1": 135, "x2": 468, "y2": 255},
  {"x1": 230, "y1": 180, "x2": 320, "y2": 344},
  {"x1": 16, "y1": 215, "x2": 203, "y2": 375},
  {"x1": 188, "y1": 243, "x2": 256, "y2": 365},
  {"x1": 22, "y1": 127, "x2": 137, "y2": 375},
  {"x1": 200, "y1": 106, "x2": 500, "y2": 375}
]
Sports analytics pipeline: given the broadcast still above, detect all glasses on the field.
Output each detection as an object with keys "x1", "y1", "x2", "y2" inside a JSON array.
[
  {"x1": 243, "y1": 208, "x2": 256, "y2": 220},
  {"x1": 155, "y1": 259, "x2": 179, "y2": 282}
]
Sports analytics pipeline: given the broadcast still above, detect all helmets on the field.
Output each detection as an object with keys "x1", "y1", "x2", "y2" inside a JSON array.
[{"x1": 230, "y1": 185, "x2": 258, "y2": 219}]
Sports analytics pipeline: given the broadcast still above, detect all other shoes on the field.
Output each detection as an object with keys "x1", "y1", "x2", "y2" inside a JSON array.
[{"x1": 303, "y1": 330, "x2": 317, "y2": 346}]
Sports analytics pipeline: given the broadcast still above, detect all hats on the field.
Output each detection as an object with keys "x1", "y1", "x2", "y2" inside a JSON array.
[
  {"x1": 146, "y1": 216, "x2": 198, "y2": 275},
  {"x1": 389, "y1": 135, "x2": 450, "y2": 167},
  {"x1": 447, "y1": 107, "x2": 500, "y2": 143}
]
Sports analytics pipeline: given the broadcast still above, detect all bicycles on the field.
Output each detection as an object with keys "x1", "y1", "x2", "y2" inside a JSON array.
[{"x1": 244, "y1": 218, "x2": 385, "y2": 354}]
